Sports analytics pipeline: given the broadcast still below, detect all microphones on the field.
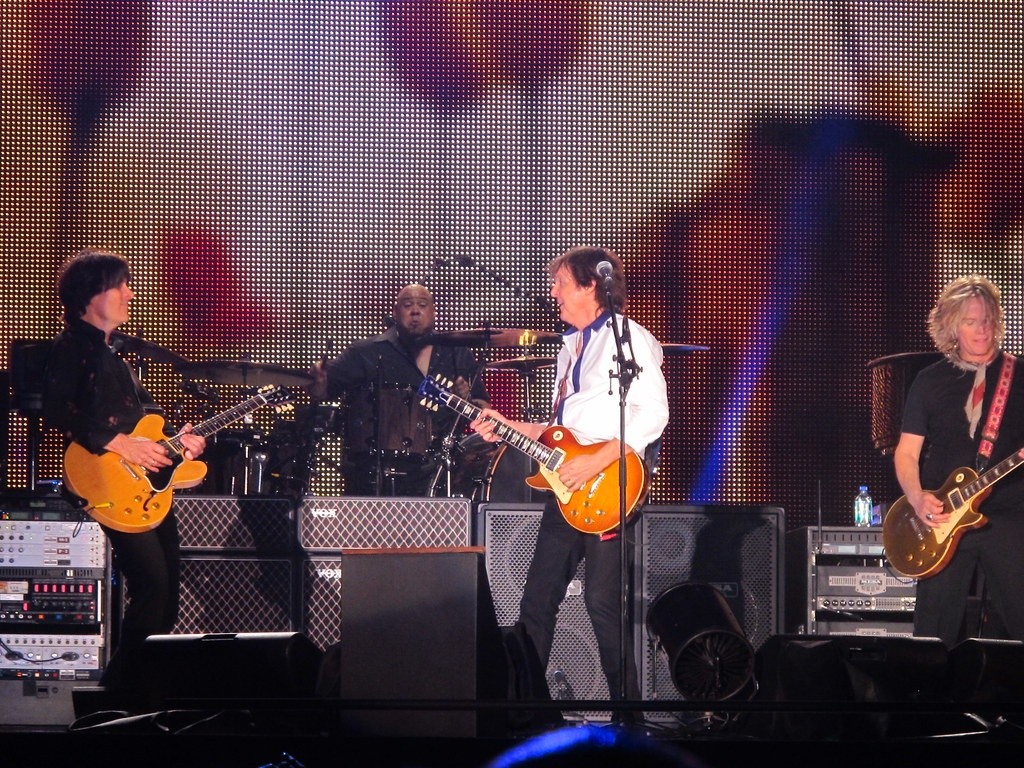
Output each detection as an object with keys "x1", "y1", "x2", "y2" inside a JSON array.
[
  {"x1": 595, "y1": 261, "x2": 613, "y2": 282},
  {"x1": 553, "y1": 670, "x2": 567, "y2": 690}
]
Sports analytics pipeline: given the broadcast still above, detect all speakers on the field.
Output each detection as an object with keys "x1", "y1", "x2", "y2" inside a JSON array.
[
  {"x1": 753, "y1": 633, "x2": 1024, "y2": 711},
  {"x1": 120, "y1": 495, "x2": 788, "y2": 725}
]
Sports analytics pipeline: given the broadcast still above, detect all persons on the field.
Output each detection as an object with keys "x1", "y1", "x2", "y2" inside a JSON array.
[
  {"x1": 306, "y1": 283, "x2": 491, "y2": 497},
  {"x1": 40, "y1": 247, "x2": 207, "y2": 692},
  {"x1": 469, "y1": 248, "x2": 670, "y2": 724},
  {"x1": 894, "y1": 273, "x2": 1023, "y2": 650}
]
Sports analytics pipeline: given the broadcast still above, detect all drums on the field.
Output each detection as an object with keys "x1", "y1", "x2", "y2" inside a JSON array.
[
  {"x1": 182, "y1": 424, "x2": 265, "y2": 497},
  {"x1": 429, "y1": 426, "x2": 551, "y2": 507}
]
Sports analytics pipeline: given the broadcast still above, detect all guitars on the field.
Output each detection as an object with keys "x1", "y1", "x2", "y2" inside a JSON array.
[
  {"x1": 882, "y1": 447, "x2": 1024, "y2": 579},
  {"x1": 62, "y1": 382, "x2": 298, "y2": 533},
  {"x1": 417, "y1": 373, "x2": 650, "y2": 535}
]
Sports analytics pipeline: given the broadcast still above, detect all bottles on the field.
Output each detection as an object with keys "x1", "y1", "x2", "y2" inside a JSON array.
[{"x1": 854, "y1": 486, "x2": 873, "y2": 527}]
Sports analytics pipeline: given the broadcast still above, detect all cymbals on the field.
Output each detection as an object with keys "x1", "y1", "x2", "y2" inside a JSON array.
[
  {"x1": 107, "y1": 329, "x2": 188, "y2": 365},
  {"x1": 657, "y1": 342, "x2": 712, "y2": 355},
  {"x1": 485, "y1": 355, "x2": 558, "y2": 370},
  {"x1": 427, "y1": 327, "x2": 560, "y2": 346},
  {"x1": 172, "y1": 359, "x2": 314, "y2": 388}
]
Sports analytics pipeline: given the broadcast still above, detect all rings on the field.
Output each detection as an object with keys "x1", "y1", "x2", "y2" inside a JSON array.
[
  {"x1": 568, "y1": 479, "x2": 574, "y2": 485},
  {"x1": 927, "y1": 513, "x2": 934, "y2": 519},
  {"x1": 150, "y1": 459, "x2": 155, "y2": 466}
]
